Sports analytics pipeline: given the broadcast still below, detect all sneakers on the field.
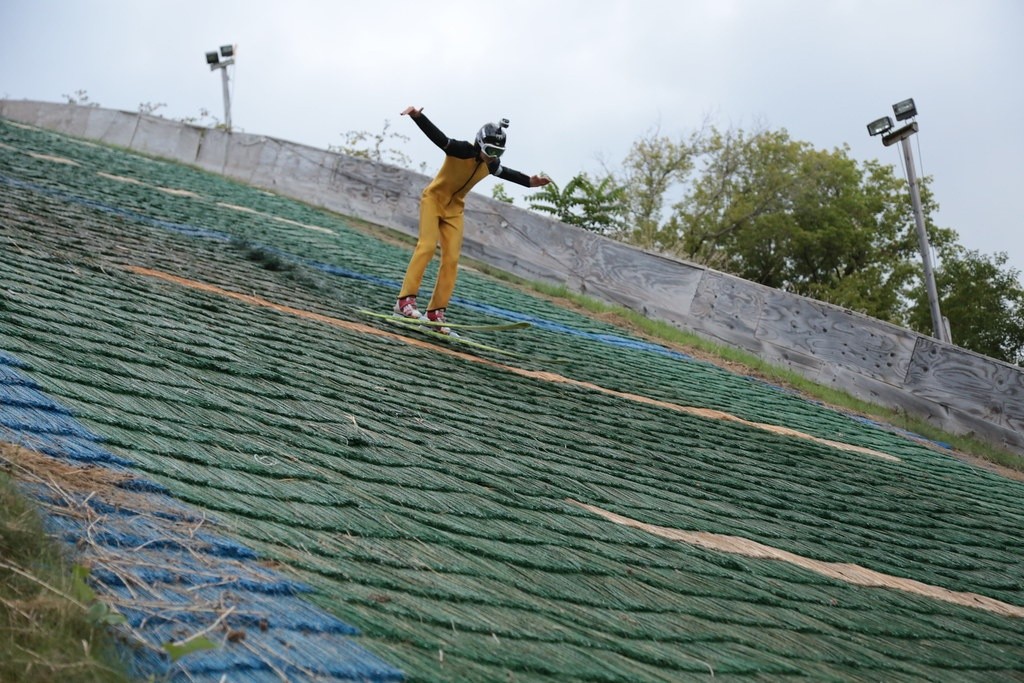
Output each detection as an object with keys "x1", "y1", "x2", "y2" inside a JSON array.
[
  {"x1": 424, "y1": 308, "x2": 450, "y2": 334},
  {"x1": 394, "y1": 295, "x2": 422, "y2": 318}
]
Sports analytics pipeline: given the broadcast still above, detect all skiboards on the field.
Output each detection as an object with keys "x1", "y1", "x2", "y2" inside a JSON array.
[{"x1": 364, "y1": 306, "x2": 533, "y2": 363}]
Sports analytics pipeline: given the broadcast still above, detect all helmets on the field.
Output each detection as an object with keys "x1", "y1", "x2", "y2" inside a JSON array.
[{"x1": 474, "y1": 118, "x2": 509, "y2": 151}]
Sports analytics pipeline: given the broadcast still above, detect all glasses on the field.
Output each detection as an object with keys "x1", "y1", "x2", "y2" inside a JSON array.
[{"x1": 481, "y1": 143, "x2": 507, "y2": 158}]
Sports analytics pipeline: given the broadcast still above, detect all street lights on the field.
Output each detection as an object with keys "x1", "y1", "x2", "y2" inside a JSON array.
[
  {"x1": 865, "y1": 97, "x2": 954, "y2": 344},
  {"x1": 205, "y1": 44, "x2": 236, "y2": 133}
]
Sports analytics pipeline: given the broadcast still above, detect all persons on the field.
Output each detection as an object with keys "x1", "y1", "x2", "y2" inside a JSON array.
[{"x1": 394, "y1": 106, "x2": 551, "y2": 335}]
